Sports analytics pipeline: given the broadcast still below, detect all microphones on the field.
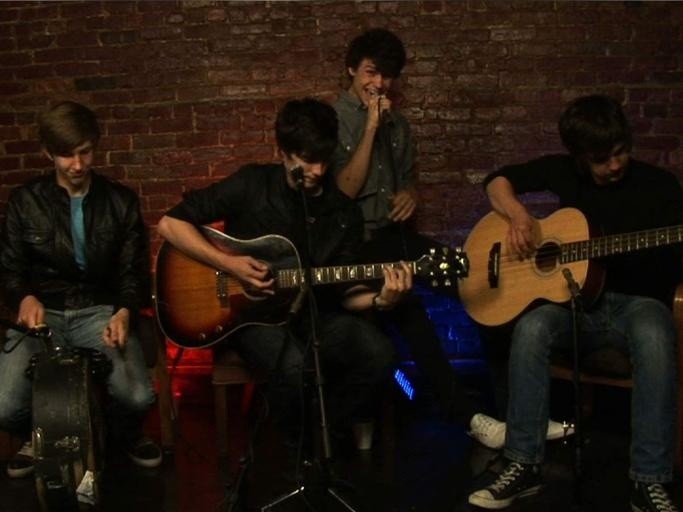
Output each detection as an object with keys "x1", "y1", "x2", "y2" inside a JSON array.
[
  {"x1": 562, "y1": 268, "x2": 584, "y2": 306},
  {"x1": 373, "y1": 94, "x2": 396, "y2": 130},
  {"x1": 42, "y1": 327, "x2": 59, "y2": 355},
  {"x1": 290, "y1": 164, "x2": 305, "y2": 187}
]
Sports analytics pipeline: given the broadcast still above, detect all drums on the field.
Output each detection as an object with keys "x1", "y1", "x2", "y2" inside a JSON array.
[{"x1": 33, "y1": 345, "x2": 111, "y2": 511}]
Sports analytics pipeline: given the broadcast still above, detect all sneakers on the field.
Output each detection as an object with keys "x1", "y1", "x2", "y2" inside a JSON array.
[
  {"x1": 470, "y1": 412, "x2": 506, "y2": 449},
  {"x1": 468, "y1": 462, "x2": 541, "y2": 510},
  {"x1": 546, "y1": 418, "x2": 574, "y2": 440},
  {"x1": 350, "y1": 420, "x2": 373, "y2": 451},
  {"x1": 115, "y1": 435, "x2": 163, "y2": 468},
  {"x1": 7, "y1": 440, "x2": 35, "y2": 478},
  {"x1": 631, "y1": 481, "x2": 677, "y2": 512}
]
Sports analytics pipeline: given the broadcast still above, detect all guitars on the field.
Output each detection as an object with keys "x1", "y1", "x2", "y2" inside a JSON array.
[
  {"x1": 457, "y1": 210, "x2": 683, "y2": 328},
  {"x1": 154, "y1": 224, "x2": 469, "y2": 350}
]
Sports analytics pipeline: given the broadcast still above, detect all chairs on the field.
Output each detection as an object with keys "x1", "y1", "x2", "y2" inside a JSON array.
[
  {"x1": 550, "y1": 282, "x2": 682, "y2": 389},
  {"x1": 140, "y1": 273, "x2": 257, "y2": 487}
]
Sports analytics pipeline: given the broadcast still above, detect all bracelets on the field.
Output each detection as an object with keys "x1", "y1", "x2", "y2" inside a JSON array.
[{"x1": 372, "y1": 293, "x2": 394, "y2": 311}]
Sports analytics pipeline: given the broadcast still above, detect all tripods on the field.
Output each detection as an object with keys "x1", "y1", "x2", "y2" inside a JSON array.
[{"x1": 259, "y1": 188, "x2": 356, "y2": 512}]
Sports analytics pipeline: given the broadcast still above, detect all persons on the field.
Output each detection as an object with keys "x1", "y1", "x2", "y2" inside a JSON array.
[
  {"x1": 328, "y1": 28, "x2": 575, "y2": 450},
  {"x1": 0, "y1": 101, "x2": 161, "y2": 478},
  {"x1": 156, "y1": 97, "x2": 412, "y2": 512},
  {"x1": 469, "y1": 94, "x2": 683, "y2": 512}
]
